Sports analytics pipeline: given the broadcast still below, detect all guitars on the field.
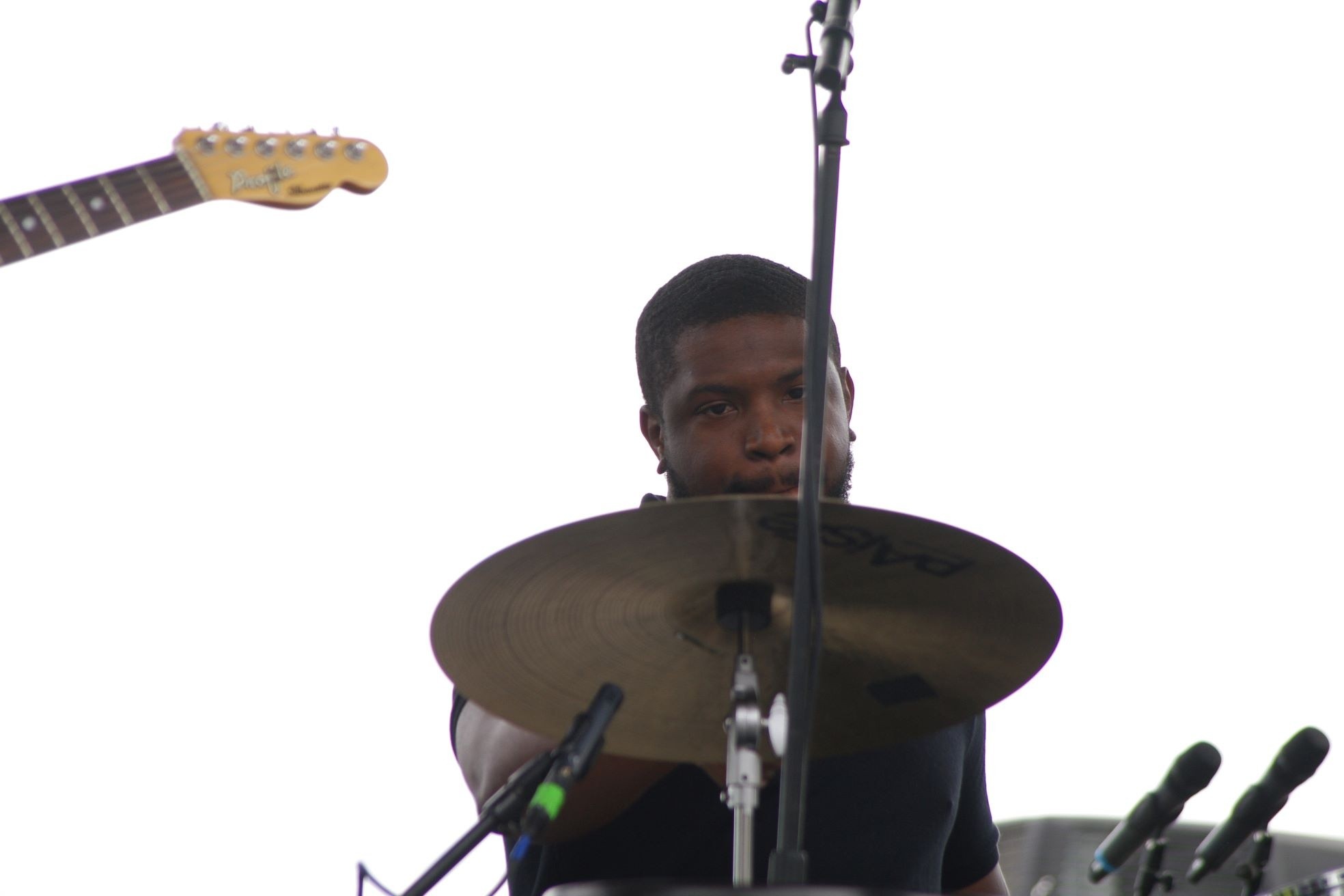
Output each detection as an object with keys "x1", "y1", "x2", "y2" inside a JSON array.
[{"x1": 0, "y1": 114, "x2": 395, "y2": 267}]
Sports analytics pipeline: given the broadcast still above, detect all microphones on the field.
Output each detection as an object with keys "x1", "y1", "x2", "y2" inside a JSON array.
[
  {"x1": 1086, "y1": 742, "x2": 1221, "y2": 884},
  {"x1": 1184, "y1": 728, "x2": 1330, "y2": 884},
  {"x1": 507, "y1": 683, "x2": 626, "y2": 866}
]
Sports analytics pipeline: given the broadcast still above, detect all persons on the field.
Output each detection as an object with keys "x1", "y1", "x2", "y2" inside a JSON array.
[{"x1": 449, "y1": 254, "x2": 1008, "y2": 896}]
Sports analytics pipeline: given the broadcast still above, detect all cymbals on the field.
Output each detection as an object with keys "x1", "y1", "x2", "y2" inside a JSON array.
[{"x1": 425, "y1": 492, "x2": 1065, "y2": 763}]
